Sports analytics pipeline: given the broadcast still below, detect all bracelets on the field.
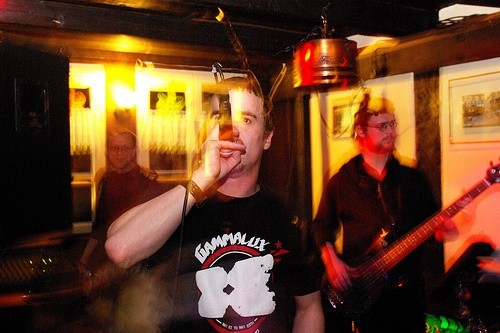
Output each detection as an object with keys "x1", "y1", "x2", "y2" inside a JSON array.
[{"x1": 179, "y1": 179, "x2": 205, "y2": 203}]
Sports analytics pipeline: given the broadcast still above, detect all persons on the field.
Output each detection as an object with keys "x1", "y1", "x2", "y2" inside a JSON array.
[
  {"x1": 77, "y1": 76, "x2": 324, "y2": 333},
  {"x1": 310, "y1": 93, "x2": 459, "y2": 332}
]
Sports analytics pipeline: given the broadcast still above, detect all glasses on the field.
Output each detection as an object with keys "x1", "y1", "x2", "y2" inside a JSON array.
[
  {"x1": 366, "y1": 121, "x2": 397, "y2": 131},
  {"x1": 108, "y1": 145, "x2": 133, "y2": 153}
]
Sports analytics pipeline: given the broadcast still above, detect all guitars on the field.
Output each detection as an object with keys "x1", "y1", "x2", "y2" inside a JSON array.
[{"x1": 316, "y1": 156, "x2": 500, "y2": 333}]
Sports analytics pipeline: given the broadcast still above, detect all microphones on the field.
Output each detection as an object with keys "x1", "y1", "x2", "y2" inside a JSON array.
[{"x1": 218, "y1": 90, "x2": 236, "y2": 158}]
